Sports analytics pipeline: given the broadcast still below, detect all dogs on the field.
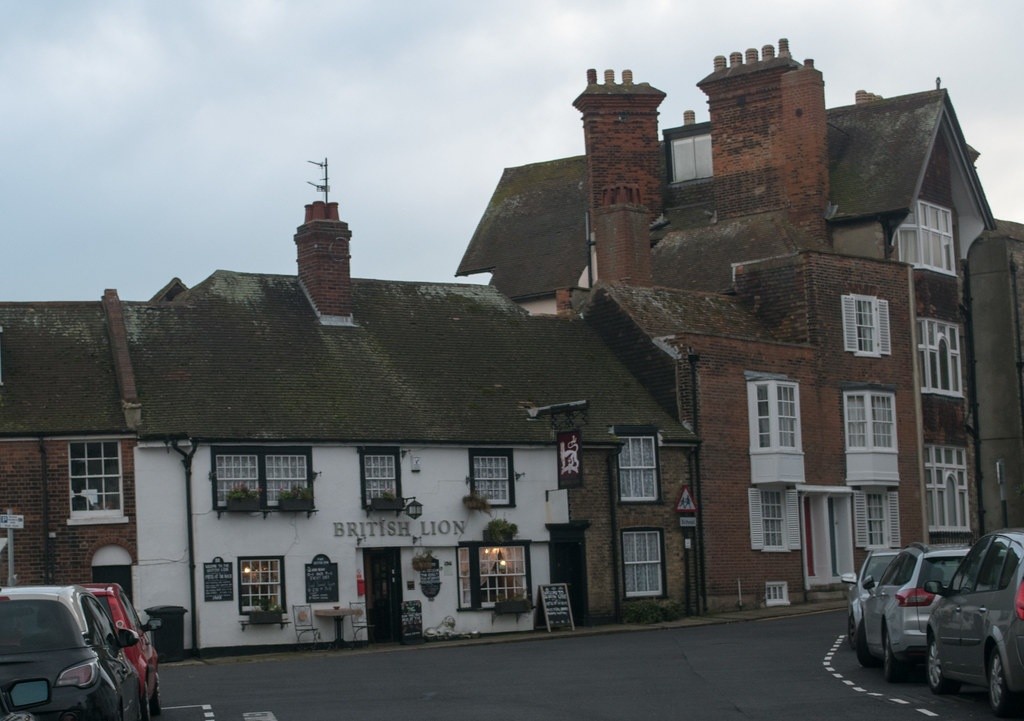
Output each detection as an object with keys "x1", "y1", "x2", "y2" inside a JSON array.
[{"x1": 425, "y1": 616, "x2": 465, "y2": 637}]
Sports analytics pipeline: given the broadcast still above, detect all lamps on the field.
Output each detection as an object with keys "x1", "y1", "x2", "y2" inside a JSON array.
[{"x1": 404, "y1": 497, "x2": 423, "y2": 519}]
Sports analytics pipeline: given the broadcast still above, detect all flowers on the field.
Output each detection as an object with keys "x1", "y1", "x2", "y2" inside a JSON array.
[
  {"x1": 276, "y1": 484, "x2": 314, "y2": 500},
  {"x1": 249, "y1": 599, "x2": 283, "y2": 611},
  {"x1": 226, "y1": 482, "x2": 259, "y2": 502},
  {"x1": 383, "y1": 486, "x2": 398, "y2": 501}
]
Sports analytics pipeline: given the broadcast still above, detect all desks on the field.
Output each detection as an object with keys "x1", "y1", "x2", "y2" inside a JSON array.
[{"x1": 314, "y1": 609, "x2": 364, "y2": 652}]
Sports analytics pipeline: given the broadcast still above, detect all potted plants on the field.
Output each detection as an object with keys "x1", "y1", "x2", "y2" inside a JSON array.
[
  {"x1": 483, "y1": 519, "x2": 519, "y2": 543},
  {"x1": 495, "y1": 590, "x2": 531, "y2": 614},
  {"x1": 463, "y1": 492, "x2": 493, "y2": 516},
  {"x1": 414, "y1": 555, "x2": 431, "y2": 571}
]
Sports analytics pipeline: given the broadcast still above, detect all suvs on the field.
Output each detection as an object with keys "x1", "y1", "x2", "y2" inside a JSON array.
[{"x1": 853, "y1": 540, "x2": 1007, "y2": 685}]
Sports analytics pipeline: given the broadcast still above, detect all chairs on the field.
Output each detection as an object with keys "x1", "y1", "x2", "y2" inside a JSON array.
[
  {"x1": 292, "y1": 605, "x2": 321, "y2": 651},
  {"x1": 349, "y1": 602, "x2": 376, "y2": 651}
]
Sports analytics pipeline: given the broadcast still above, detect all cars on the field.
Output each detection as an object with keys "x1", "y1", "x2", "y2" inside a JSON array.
[
  {"x1": 0, "y1": 585, "x2": 145, "y2": 721},
  {"x1": 921, "y1": 526, "x2": 1024, "y2": 721},
  {"x1": 840, "y1": 548, "x2": 901, "y2": 650},
  {"x1": 76, "y1": 582, "x2": 164, "y2": 721}
]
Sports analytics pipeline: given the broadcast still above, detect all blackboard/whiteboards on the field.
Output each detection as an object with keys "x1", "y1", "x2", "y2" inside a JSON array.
[
  {"x1": 401, "y1": 601, "x2": 422, "y2": 634},
  {"x1": 420, "y1": 555, "x2": 440, "y2": 598},
  {"x1": 534, "y1": 583, "x2": 573, "y2": 626},
  {"x1": 305, "y1": 554, "x2": 339, "y2": 603},
  {"x1": 203, "y1": 557, "x2": 234, "y2": 602}
]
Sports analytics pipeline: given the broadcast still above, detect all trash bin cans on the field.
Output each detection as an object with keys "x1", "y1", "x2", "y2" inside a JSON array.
[{"x1": 143, "y1": 605, "x2": 189, "y2": 663}]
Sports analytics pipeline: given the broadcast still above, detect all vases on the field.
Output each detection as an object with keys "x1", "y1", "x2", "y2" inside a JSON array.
[
  {"x1": 278, "y1": 500, "x2": 314, "y2": 510},
  {"x1": 226, "y1": 502, "x2": 260, "y2": 512},
  {"x1": 249, "y1": 611, "x2": 284, "y2": 622},
  {"x1": 371, "y1": 498, "x2": 404, "y2": 510}
]
